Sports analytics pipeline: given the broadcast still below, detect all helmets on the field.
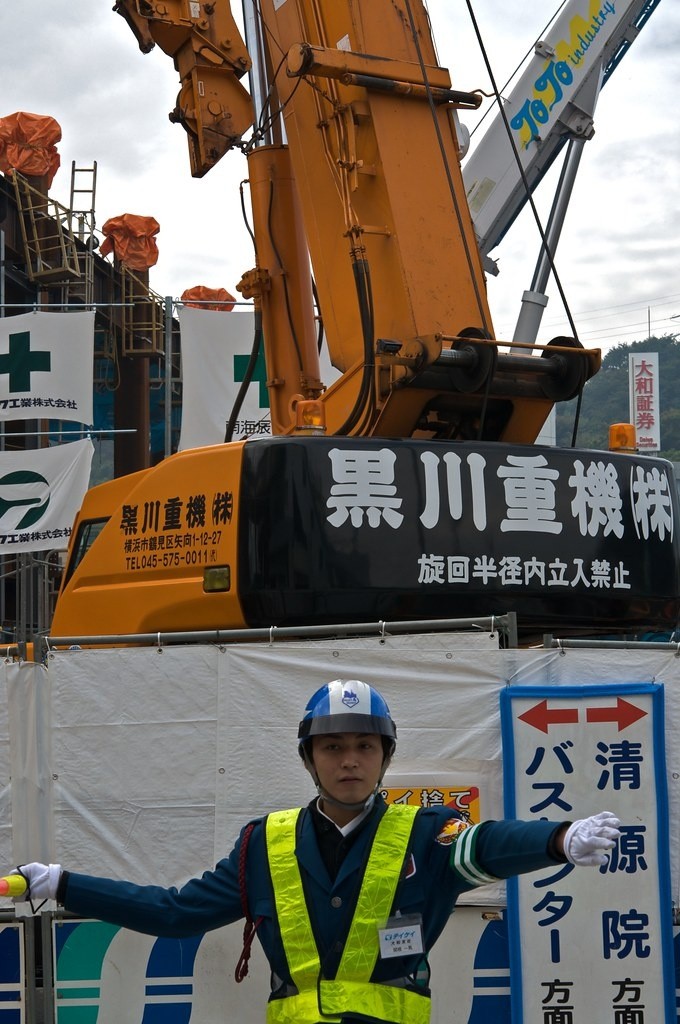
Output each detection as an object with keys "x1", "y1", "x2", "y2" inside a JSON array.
[{"x1": 297, "y1": 679, "x2": 398, "y2": 786}]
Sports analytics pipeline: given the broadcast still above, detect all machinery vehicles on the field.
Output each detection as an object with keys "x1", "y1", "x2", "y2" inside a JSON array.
[{"x1": 33, "y1": 0, "x2": 680, "y2": 648}]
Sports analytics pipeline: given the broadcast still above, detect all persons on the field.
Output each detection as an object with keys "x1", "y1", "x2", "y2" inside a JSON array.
[{"x1": 8, "y1": 681, "x2": 621, "y2": 1024}]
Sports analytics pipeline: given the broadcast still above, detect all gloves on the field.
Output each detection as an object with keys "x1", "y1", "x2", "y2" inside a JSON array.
[
  {"x1": 9, "y1": 862, "x2": 60, "y2": 902},
  {"x1": 563, "y1": 812, "x2": 620, "y2": 866}
]
